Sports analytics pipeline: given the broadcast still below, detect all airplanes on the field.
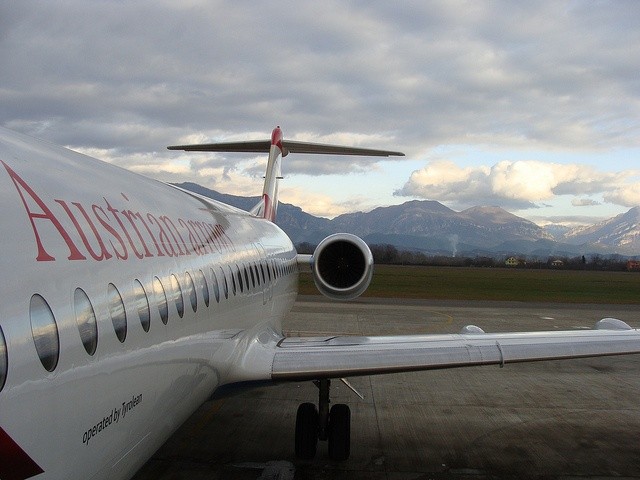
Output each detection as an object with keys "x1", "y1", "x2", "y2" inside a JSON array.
[{"x1": 0, "y1": 121, "x2": 640, "y2": 480}]
